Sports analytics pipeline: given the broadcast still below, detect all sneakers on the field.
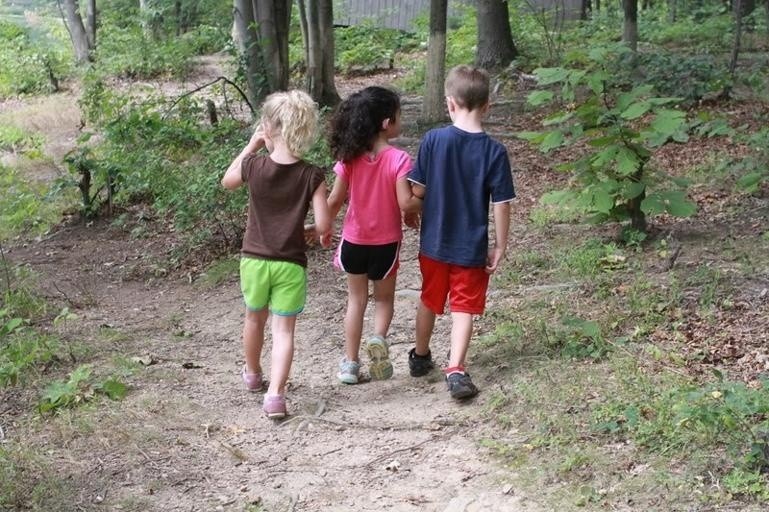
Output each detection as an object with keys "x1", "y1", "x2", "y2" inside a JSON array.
[
  {"x1": 366, "y1": 337, "x2": 393, "y2": 380},
  {"x1": 445, "y1": 371, "x2": 477, "y2": 400},
  {"x1": 262, "y1": 393, "x2": 286, "y2": 419},
  {"x1": 337, "y1": 355, "x2": 362, "y2": 385},
  {"x1": 409, "y1": 347, "x2": 435, "y2": 376},
  {"x1": 240, "y1": 363, "x2": 263, "y2": 392}
]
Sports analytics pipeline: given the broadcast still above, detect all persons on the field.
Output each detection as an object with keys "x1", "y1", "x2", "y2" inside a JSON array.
[
  {"x1": 406, "y1": 62, "x2": 517, "y2": 402},
  {"x1": 319, "y1": 83, "x2": 426, "y2": 385},
  {"x1": 220, "y1": 88, "x2": 335, "y2": 420}
]
